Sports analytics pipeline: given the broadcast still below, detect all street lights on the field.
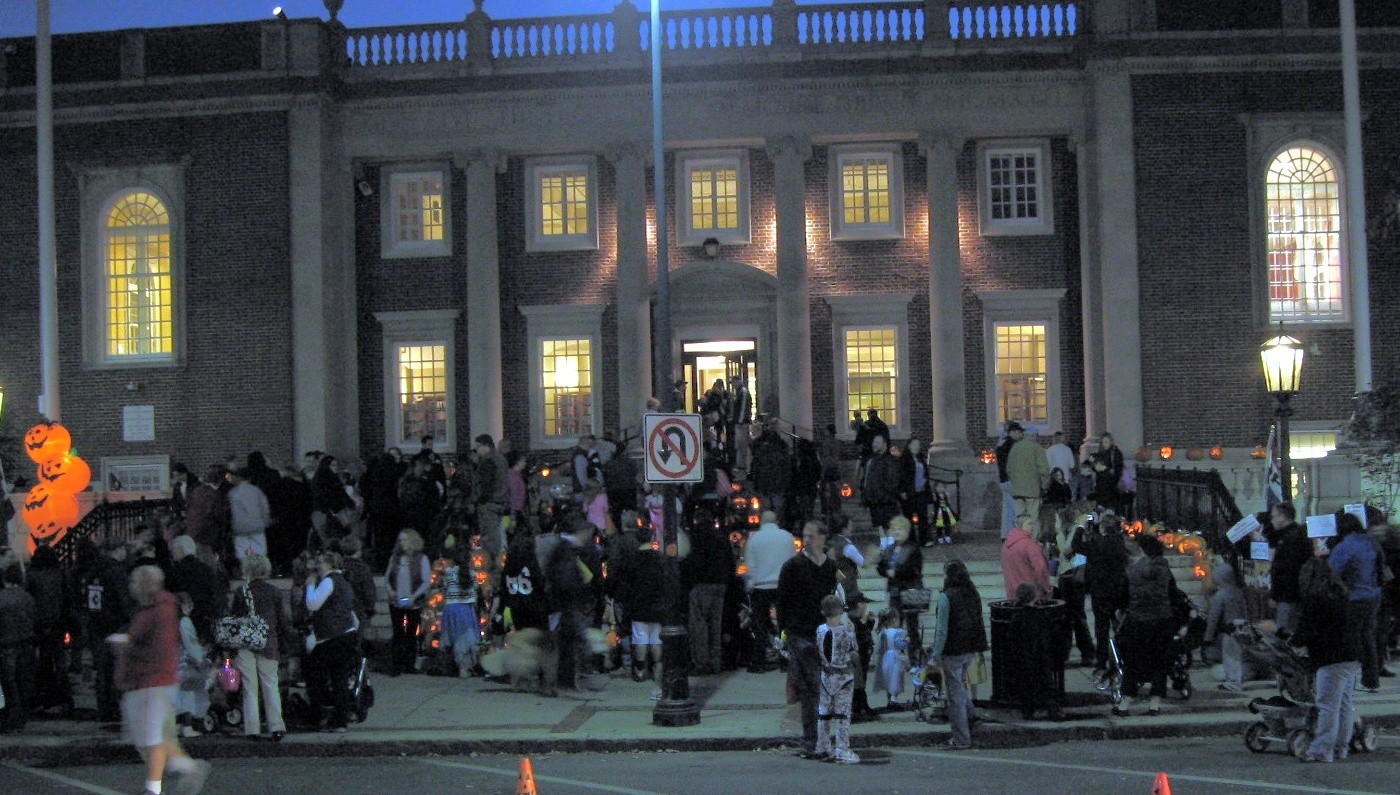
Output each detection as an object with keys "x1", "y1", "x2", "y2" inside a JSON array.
[{"x1": 1257, "y1": 321, "x2": 1307, "y2": 523}]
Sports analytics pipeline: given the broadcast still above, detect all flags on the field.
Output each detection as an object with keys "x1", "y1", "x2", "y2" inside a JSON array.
[{"x1": 1264, "y1": 433, "x2": 1284, "y2": 512}]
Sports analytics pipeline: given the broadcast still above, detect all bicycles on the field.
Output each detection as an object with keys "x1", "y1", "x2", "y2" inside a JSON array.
[{"x1": 913, "y1": 658, "x2": 948, "y2": 721}]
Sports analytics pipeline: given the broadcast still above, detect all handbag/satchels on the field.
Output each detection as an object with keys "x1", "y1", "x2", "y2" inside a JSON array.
[
  {"x1": 211, "y1": 613, "x2": 269, "y2": 652},
  {"x1": 1378, "y1": 561, "x2": 1394, "y2": 586}
]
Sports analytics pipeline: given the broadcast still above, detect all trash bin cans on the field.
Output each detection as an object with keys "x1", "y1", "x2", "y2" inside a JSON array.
[{"x1": 987, "y1": 598, "x2": 1067, "y2": 707}]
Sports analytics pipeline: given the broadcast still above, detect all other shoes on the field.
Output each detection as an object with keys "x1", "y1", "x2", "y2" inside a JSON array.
[
  {"x1": 182, "y1": 725, "x2": 201, "y2": 738},
  {"x1": 834, "y1": 747, "x2": 860, "y2": 764},
  {"x1": 1355, "y1": 682, "x2": 1379, "y2": 693},
  {"x1": 634, "y1": 666, "x2": 646, "y2": 683},
  {"x1": 940, "y1": 738, "x2": 971, "y2": 750},
  {"x1": 1379, "y1": 667, "x2": 1397, "y2": 678},
  {"x1": 175, "y1": 723, "x2": 184, "y2": 735}
]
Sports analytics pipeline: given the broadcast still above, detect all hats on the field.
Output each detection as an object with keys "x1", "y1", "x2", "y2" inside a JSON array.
[{"x1": 1008, "y1": 422, "x2": 1025, "y2": 432}]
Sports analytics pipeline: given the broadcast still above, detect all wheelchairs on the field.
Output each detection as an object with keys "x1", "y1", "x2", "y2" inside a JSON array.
[{"x1": 1093, "y1": 604, "x2": 1196, "y2": 706}]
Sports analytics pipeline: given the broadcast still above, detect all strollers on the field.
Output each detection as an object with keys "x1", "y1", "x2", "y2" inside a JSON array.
[
  {"x1": 280, "y1": 647, "x2": 374, "y2": 728},
  {"x1": 1230, "y1": 617, "x2": 1383, "y2": 761},
  {"x1": 193, "y1": 638, "x2": 247, "y2": 732}
]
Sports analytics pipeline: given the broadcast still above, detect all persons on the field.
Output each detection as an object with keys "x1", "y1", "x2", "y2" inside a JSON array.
[
  {"x1": 1, "y1": 448, "x2": 378, "y2": 740},
  {"x1": 357, "y1": 409, "x2": 988, "y2": 764},
  {"x1": 641, "y1": 376, "x2": 752, "y2": 479},
  {"x1": 112, "y1": 563, "x2": 209, "y2": 795},
  {"x1": 994, "y1": 421, "x2": 1136, "y2": 540},
  {"x1": 1003, "y1": 508, "x2": 1177, "y2": 716},
  {"x1": 1202, "y1": 500, "x2": 1399, "y2": 762}
]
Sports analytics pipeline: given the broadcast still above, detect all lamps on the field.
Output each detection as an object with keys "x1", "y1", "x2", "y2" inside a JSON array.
[
  {"x1": 127, "y1": 381, "x2": 140, "y2": 391},
  {"x1": 272, "y1": 7, "x2": 288, "y2": 21},
  {"x1": 703, "y1": 238, "x2": 721, "y2": 261}
]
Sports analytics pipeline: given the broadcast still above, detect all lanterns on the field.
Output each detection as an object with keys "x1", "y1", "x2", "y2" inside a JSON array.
[
  {"x1": 1251, "y1": 446, "x2": 1267, "y2": 459},
  {"x1": 1160, "y1": 445, "x2": 1174, "y2": 461},
  {"x1": 980, "y1": 449, "x2": 996, "y2": 464},
  {"x1": 1118, "y1": 517, "x2": 1217, "y2": 591},
  {"x1": 21, "y1": 417, "x2": 91, "y2": 554},
  {"x1": 1209, "y1": 446, "x2": 1224, "y2": 460}
]
